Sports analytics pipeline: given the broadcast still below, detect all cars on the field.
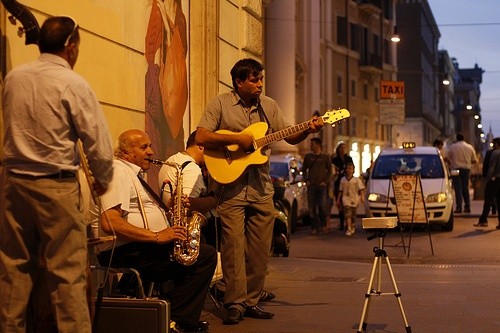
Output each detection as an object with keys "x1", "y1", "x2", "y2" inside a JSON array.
[
  {"x1": 361, "y1": 140, "x2": 460, "y2": 232},
  {"x1": 269, "y1": 155, "x2": 309, "y2": 234}
]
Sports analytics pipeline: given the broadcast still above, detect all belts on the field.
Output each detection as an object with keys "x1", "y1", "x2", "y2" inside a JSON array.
[{"x1": 45, "y1": 169, "x2": 76, "y2": 178}]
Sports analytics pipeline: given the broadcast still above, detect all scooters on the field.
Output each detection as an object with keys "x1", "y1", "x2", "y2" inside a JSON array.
[{"x1": 270, "y1": 158, "x2": 303, "y2": 259}]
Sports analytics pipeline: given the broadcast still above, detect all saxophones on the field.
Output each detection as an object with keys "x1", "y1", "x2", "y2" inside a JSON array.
[{"x1": 146, "y1": 157, "x2": 207, "y2": 266}]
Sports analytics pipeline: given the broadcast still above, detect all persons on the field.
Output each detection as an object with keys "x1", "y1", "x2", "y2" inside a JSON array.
[
  {"x1": 336, "y1": 162, "x2": 367, "y2": 237},
  {"x1": 88, "y1": 128, "x2": 218, "y2": 333},
  {"x1": 473, "y1": 137, "x2": 500, "y2": 229},
  {"x1": 144, "y1": 0, "x2": 188, "y2": 162},
  {"x1": 158, "y1": 130, "x2": 277, "y2": 305},
  {"x1": 302, "y1": 137, "x2": 333, "y2": 234},
  {"x1": 441, "y1": 133, "x2": 477, "y2": 214},
  {"x1": 193, "y1": 58, "x2": 324, "y2": 324},
  {"x1": 0, "y1": 16, "x2": 113, "y2": 333},
  {"x1": 331, "y1": 143, "x2": 355, "y2": 231}
]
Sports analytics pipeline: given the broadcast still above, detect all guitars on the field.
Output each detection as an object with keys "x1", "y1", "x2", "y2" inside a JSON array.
[{"x1": 203, "y1": 107, "x2": 351, "y2": 185}]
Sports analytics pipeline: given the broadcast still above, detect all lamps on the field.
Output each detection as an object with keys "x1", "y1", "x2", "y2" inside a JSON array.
[
  {"x1": 391, "y1": 26, "x2": 400, "y2": 42},
  {"x1": 443, "y1": 75, "x2": 450, "y2": 86}
]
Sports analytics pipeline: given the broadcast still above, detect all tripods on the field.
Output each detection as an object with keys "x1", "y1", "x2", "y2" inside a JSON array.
[{"x1": 357, "y1": 229, "x2": 412, "y2": 333}]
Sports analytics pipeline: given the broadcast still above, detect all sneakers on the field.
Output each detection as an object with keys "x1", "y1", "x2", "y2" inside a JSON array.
[{"x1": 257, "y1": 289, "x2": 276, "y2": 301}]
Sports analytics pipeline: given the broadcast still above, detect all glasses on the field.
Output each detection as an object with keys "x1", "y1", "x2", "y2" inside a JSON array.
[{"x1": 63, "y1": 16, "x2": 79, "y2": 47}]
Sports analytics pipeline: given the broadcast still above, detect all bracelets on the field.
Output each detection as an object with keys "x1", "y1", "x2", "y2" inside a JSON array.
[{"x1": 156, "y1": 232, "x2": 158, "y2": 242}]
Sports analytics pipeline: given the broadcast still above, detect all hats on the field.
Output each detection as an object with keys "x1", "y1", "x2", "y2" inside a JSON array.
[
  {"x1": 457, "y1": 132, "x2": 464, "y2": 139},
  {"x1": 336, "y1": 140, "x2": 347, "y2": 150},
  {"x1": 433, "y1": 139, "x2": 443, "y2": 145},
  {"x1": 493, "y1": 138, "x2": 500, "y2": 143}
]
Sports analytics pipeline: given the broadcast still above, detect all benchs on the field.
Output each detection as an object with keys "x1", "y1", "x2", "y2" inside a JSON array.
[{"x1": 398, "y1": 160, "x2": 418, "y2": 174}]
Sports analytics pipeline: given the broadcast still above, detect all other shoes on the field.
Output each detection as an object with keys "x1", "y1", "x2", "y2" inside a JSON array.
[
  {"x1": 311, "y1": 229, "x2": 317, "y2": 234},
  {"x1": 496, "y1": 225, "x2": 500, "y2": 229},
  {"x1": 199, "y1": 320, "x2": 209, "y2": 329},
  {"x1": 473, "y1": 222, "x2": 488, "y2": 227},
  {"x1": 454, "y1": 207, "x2": 462, "y2": 213},
  {"x1": 336, "y1": 227, "x2": 344, "y2": 233},
  {"x1": 464, "y1": 206, "x2": 470, "y2": 212},
  {"x1": 317, "y1": 226, "x2": 331, "y2": 234},
  {"x1": 352, "y1": 224, "x2": 356, "y2": 235},
  {"x1": 345, "y1": 225, "x2": 352, "y2": 237}
]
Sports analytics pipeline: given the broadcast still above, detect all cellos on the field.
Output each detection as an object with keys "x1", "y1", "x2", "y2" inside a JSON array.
[{"x1": 0, "y1": 0, "x2": 117, "y2": 326}]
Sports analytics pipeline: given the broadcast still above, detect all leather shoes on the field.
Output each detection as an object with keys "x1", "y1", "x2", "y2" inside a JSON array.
[
  {"x1": 226, "y1": 307, "x2": 243, "y2": 324},
  {"x1": 244, "y1": 304, "x2": 275, "y2": 321}
]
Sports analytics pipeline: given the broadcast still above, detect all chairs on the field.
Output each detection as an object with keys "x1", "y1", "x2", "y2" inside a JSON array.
[
  {"x1": 378, "y1": 162, "x2": 399, "y2": 175},
  {"x1": 89, "y1": 265, "x2": 147, "y2": 299},
  {"x1": 417, "y1": 158, "x2": 435, "y2": 177}
]
